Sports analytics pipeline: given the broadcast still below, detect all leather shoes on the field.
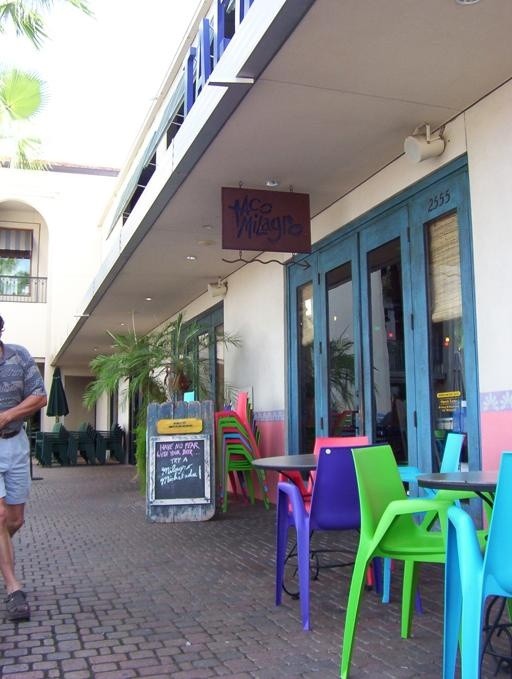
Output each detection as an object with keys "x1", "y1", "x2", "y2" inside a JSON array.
[{"x1": 4, "y1": 589, "x2": 31, "y2": 620}]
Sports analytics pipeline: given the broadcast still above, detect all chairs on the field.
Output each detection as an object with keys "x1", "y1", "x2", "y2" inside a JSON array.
[
  {"x1": 338, "y1": 442, "x2": 495, "y2": 678},
  {"x1": 213, "y1": 390, "x2": 272, "y2": 512},
  {"x1": 379, "y1": 434, "x2": 470, "y2": 603},
  {"x1": 34, "y1": 423, "x2": 126, "y2": 468},
  {"x1": 441, "y1": 450, "x2": 509, "y2": 678},
  {"x1": 275, "y1": 445, "x2": 383, "y2": 631},
  {"x1": 288, "y1": 434, "x2": 394, "y2": 590}
]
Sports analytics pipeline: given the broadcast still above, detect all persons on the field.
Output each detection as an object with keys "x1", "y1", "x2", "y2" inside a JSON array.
[{"x1": 0, "y1": 315, "x2": 49, "y2": 622}]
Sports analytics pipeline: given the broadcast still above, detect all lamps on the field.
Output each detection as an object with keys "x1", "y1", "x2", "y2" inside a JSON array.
[
  {"x1": 403, "y1": 120, "x2": 446, "y2": 163},
  {"x1": 206, "y1": 275, "x2": 228, "y2": 297}
]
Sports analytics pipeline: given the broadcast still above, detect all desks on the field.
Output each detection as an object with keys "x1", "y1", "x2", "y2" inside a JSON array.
[
  {"x1": 252, "y1": 454, "x2": 361, "y2": 601},
  {"x1": 417, "y1": 469, "x2": 511, "y2": 678}
]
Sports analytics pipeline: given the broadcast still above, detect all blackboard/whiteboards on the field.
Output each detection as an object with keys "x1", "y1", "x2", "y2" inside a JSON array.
[{"x1": 149, "y1": 435, "x2": 211, "y2": 505}]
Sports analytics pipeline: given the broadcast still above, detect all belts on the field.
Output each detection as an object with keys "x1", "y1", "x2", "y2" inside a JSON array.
[{"x1": 0, "y1": 426, "x2": 21, "y2": 438}]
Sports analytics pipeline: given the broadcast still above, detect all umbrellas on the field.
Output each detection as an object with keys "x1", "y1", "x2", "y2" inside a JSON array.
[{"x1": 45, "y1": 366, "x2": 70, "y2": 423}]
